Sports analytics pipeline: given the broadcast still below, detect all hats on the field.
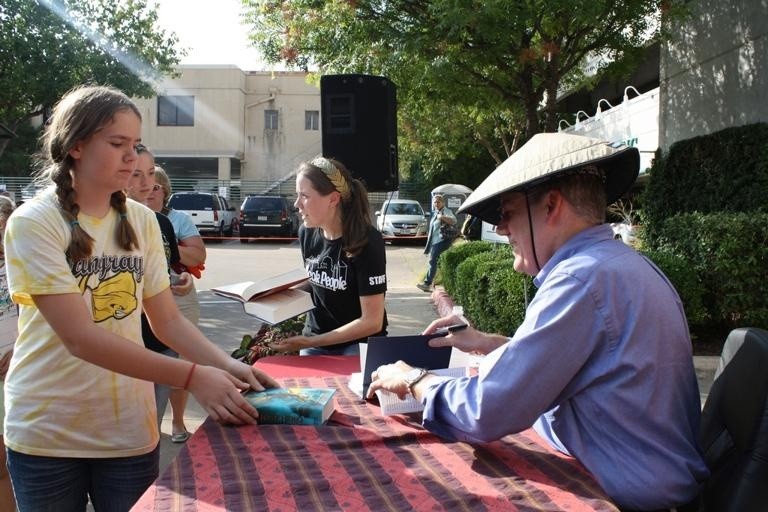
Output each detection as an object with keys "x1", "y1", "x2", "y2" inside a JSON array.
[{"x1": 455, "y1": 131, "x2": 641, "y2": 225}]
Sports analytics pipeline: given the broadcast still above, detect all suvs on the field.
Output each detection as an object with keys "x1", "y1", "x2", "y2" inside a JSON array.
[
  {"x1": 238, "y1": 194, "x2": 299, "y2": 244},
  {"x1": 166, "y1": 191, "x2": 236, "y2": 244}
]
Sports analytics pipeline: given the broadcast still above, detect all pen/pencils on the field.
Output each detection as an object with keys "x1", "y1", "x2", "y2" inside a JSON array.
[{"x1": 431, "y1": 324, "x2": 468, "y2": 335}]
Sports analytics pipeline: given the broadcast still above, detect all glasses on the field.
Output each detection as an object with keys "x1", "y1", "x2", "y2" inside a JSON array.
[{"x1": 152, "y1": 184, "x2": 164, "y2": 191}]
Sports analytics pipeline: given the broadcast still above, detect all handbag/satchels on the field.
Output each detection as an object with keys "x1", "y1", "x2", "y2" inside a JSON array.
[{"x1": 440, "y1": 208, "x2": 461, "y2": 239}]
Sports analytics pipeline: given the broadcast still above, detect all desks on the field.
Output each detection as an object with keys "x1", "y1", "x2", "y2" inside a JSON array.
[{"x1": 119, "y1": 345, "x2": 618, "y2": 511}]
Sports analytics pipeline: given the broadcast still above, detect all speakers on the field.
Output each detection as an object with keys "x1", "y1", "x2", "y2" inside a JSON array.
[{"x1": 321, "y1": 75, "x2": 399, "y2": 192}]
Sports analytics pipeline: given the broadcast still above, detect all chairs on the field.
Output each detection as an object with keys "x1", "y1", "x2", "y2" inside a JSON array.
[{"x1": 693, "y1": 319, "x2": 768, "y2": 510}]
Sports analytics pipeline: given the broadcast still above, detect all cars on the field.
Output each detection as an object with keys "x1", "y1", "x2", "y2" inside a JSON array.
[
  {"x1": 375, "y1": 199, "x2": 431, "y2": 246},
  {"x1": 608, "y1": 209, "x2": 645, "y2": 248},
  {"x1": 16, "y1": 179, "x2": 40, "y2": 203}
]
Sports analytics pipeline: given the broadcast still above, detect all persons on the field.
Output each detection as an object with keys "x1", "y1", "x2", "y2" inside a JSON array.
[
  {"x1": 1, "y1": 194, "x2": 21, "y2": 511},
  {"x1": 416, "y1": 193, "x2": 463, "y2": 296},
  {"x1": 118, "y1": 142, "x2": 183, "y2": 440},
  {"x1": 142, "y1": 160, "x2": 207, "y2": 446},
  {"x1": 246, "y1": 153, "x2": 393, "y2": 360},
  {"x1": 362, "y1": 167, "x2": 712, "y2": 512},
  {"x1": 2, "y1": 82, "x2": 284, "y2": 512}
]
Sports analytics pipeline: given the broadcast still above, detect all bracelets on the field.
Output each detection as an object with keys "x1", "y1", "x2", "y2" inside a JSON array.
[
  {"x1": 403, "y1": 367, "x2": 431, "y2": 402},
  {"x1": 183, "y1": 362, "x2": 199, "y2": 391}
]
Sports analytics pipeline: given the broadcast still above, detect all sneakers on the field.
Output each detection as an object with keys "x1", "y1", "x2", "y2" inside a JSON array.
[
  {"x1": 172, "y1": 420, "x2": 189, "y2": 443},
  {"x1": 416, "y1": 282, "x2": 429, "y2": 291}
]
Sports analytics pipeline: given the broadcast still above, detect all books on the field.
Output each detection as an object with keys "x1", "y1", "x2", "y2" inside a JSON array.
[
  {"x1": 346, "y1": 328, "x2": 458, "y2": 406},
  {"x1": 209, "y1": 268, "x2": 319, "y2": 329},
  {"x1": 237, "y1": 378, "x2": 341, "y2": 427}
]
